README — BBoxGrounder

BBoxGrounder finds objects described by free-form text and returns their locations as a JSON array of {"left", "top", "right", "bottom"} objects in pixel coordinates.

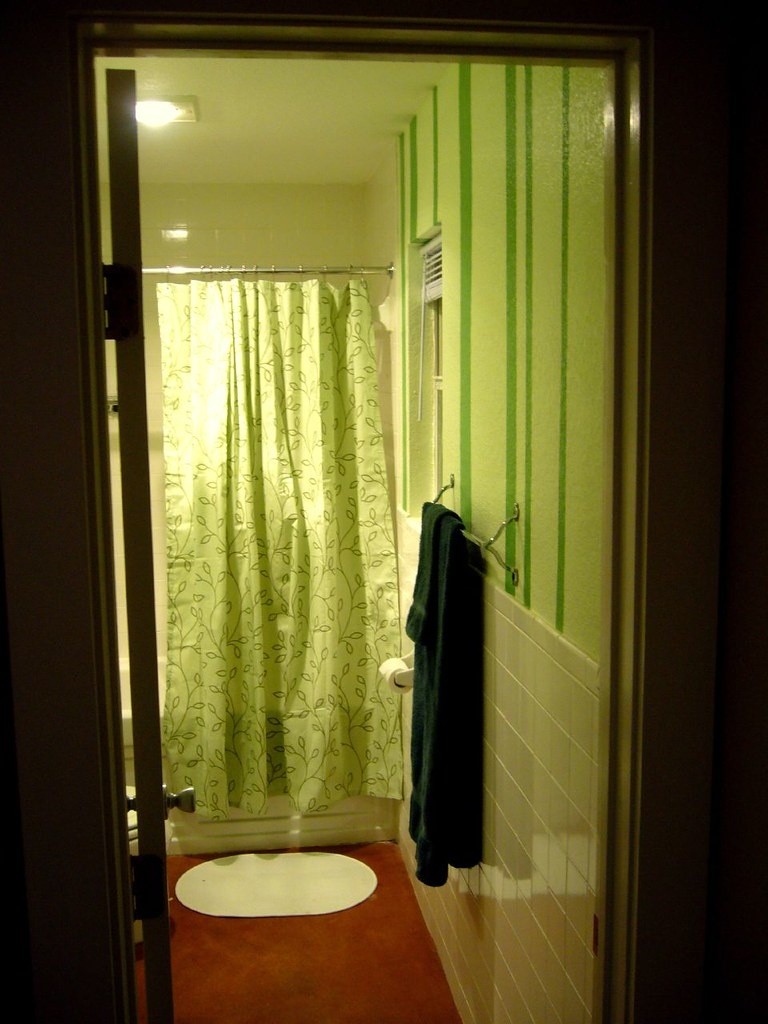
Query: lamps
[{"left": 135, "top": 96, "right": 200, "bottom": 132}]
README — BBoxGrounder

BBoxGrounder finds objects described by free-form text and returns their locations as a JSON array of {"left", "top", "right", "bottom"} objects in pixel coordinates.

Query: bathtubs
[{"left": 120, "top": 660, "right": 402, "bottom": 855}]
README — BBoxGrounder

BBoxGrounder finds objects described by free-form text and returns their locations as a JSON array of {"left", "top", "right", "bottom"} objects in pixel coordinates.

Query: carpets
[{"left": 174, "top": 852, "right": 378, "bottom": 917}]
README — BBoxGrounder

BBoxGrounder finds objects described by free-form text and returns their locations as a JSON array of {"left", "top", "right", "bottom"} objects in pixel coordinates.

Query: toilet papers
[{"left": 378, "top": 657, "right": 413, "bottom": 694}]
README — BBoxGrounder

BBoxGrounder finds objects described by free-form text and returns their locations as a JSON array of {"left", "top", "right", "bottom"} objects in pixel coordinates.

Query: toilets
[{"left": 127, "top": 782, "right": 173, "bottom": 946}]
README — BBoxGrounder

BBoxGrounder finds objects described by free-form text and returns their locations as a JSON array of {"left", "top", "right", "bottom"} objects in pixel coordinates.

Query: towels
[{"left": 404, "top": 502, "right": 487, "bottom": 888}]
